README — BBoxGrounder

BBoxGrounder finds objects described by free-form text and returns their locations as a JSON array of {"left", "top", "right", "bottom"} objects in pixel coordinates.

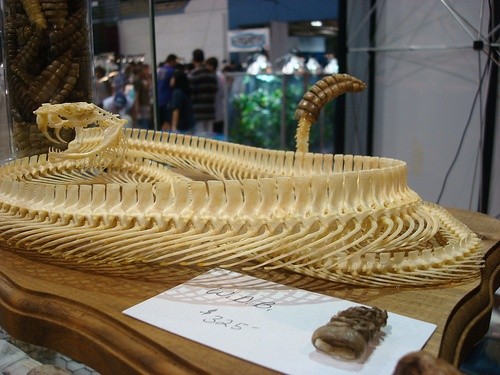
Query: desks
[{"left": 0, "top": 168, "right": 500, "bottom": 375}]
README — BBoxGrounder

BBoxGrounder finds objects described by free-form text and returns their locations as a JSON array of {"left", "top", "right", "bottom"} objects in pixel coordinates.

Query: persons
[
  {"left": 149, "top": 49, "right": 228, "bottom": 134},
  {"left": 94, "top": 64, "right": 154, "bottom": 130},
  {"left": 221, "top": 47, "right": 339, "bottom": 91}
]
